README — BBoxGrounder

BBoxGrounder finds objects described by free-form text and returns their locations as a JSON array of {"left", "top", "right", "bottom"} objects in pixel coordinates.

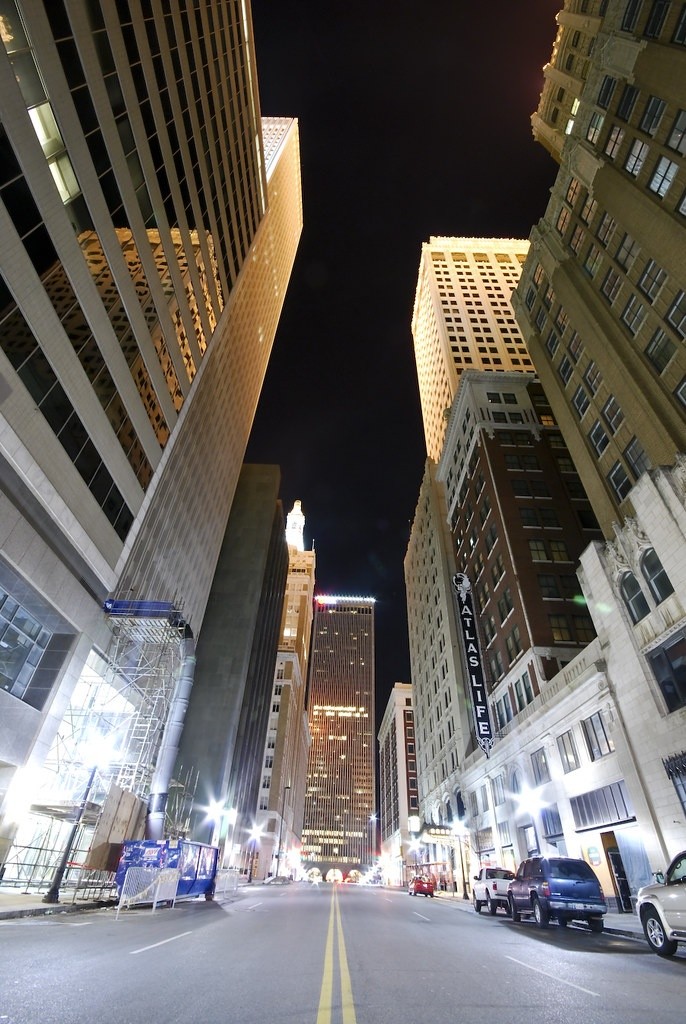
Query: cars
[
  {"left": 635, "top": 848, "right": 686, "bottom": 957},
  {"left": 407, "top": 876, "right": 434, "bottom": 897},
  {"left": 473, "top": 867, "right": 515, "bottom": 916},
  {"left": 505, "top": 856, "right": 608, "bottom": 933}
]
